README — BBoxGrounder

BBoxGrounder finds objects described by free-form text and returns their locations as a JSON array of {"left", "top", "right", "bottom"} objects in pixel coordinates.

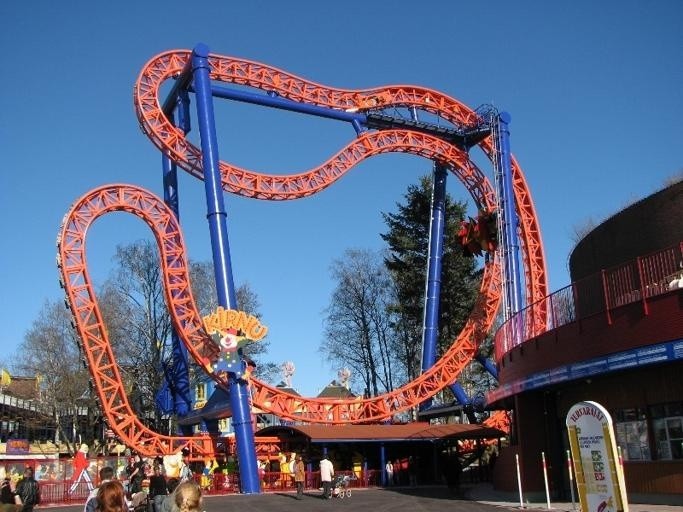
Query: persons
[
  {"left": 386, "top": 456, "right": 419, "bottom": 487},
  {"left": 440, "top": 452, "right": 464, "bottom": 500},
  {"left": 293, "top": 454, "right": 305, "bottom": 500},
  {"left": 0, "top": 464, "right": 41, "bottom": 510},
  {"left": 84, "top": 457, "right": 203, "bottom": 512},
  {"left": 319, "top": 454, "right": 334, "bottom": 500},
  {"left": 66, "top": 443, "right": 94, "bottom": 494}
]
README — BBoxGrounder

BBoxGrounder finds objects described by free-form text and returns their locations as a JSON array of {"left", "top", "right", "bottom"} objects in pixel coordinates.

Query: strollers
[{"left": 331, "top": 473, "right": 352, "bottom": 499}]
